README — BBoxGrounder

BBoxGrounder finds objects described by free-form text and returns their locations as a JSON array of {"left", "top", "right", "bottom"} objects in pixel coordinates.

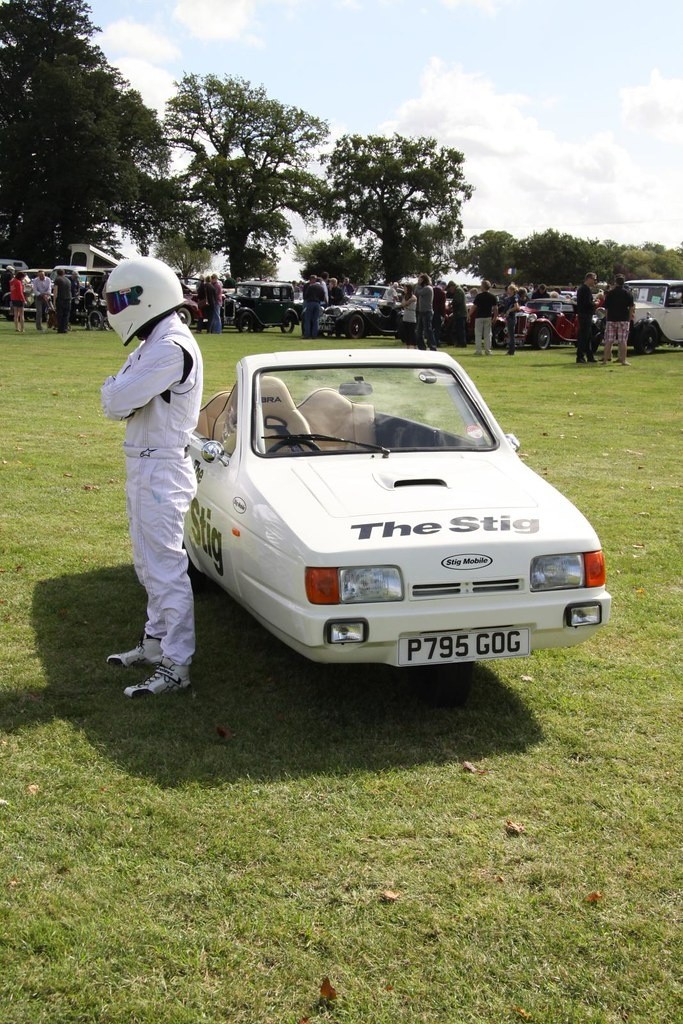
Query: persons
[
  {"left": 447, "top": 281, "right": 468, "bottom": 348},
  {"left": 386, "top": 281, "right": 399, "bottom": 301},
  {"left": 94, "top": 272, "right": 108, "bottom": 296},
  {"left": 401, "top": 282, "right": 417, "bottom": 349},
  {"left": 99, "top": 256, "right": 204, "bottom": 699},
  {"left": 84, "top": 280, "right": 101, "bottom": 330},
  {"left": 68, "top": 270, "right": 81, "bottom": 325},
  {"left": 291, "top": 272, "right": 355, "bottom": 338},
  {"left": 33, "top": 270, "right": 52, "bottom": 330},
  {"left": 600, "top": 274, "right": 634, "bottom": 366},
  {"left": 9, "top": 272, "right": 28, "bottom": 332},
  {"left": 1, "top": 266, "right": 15, "bottom": 293},
  {"left": 467, "top": 280, "right": 498, "bottom": 355},
  {"left": 415, "top": 274, "right": 438, "bottom": 351},
  {"left": 196, "top": 273, "right": 244, "bottom": 334},
  {"left": 53, "top": 269, "right": 70, "bottom": 333},
  {"left": 503, "top": 284, "right": 519, "bottom": 354},
  {"left": 433, "top": 281, "right": 449, "bottom": 347},
  {"left": 500, "top": 287, "right": 507, "bottom": 300},
  {"left": 523, "top": 282, "right": 612, "bottom": 298},
  {"left": 519, "top": 288, "right": 530, "bottom": 305},
  {"left": 576, "top": 272, "right": 600, "bottom": 364},
  {"left": 469, "top": 288, "right": 479, "bottom": 297}
]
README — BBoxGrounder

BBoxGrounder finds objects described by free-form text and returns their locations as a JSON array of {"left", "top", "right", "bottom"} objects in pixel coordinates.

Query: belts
[{"left": 122, "top": 444, "right": 190, "bottom": 460}]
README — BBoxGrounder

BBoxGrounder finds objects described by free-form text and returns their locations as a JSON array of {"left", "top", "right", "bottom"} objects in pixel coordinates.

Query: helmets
[{"left": 104, "top": 257, "right": 189, "bottom": 347}]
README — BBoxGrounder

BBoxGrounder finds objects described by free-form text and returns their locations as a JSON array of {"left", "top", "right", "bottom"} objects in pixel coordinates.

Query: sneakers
[
  {"left": 123, "top": 657, "right": 191, "bottom": 699},
  {"left": 106, "top": 632, "right": 163, "bottom": 667}
]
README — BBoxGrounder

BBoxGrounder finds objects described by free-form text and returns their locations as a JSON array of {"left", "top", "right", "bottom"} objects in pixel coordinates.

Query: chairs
[{"left": 193, "top": 375, "right": 377, "bottom": 455}]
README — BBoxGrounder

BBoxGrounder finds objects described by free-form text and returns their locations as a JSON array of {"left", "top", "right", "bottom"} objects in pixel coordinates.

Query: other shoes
[
  {"left": 406, "top": 342, "right": 466, "bottom": 351},
  {"left": 576, "top": 357, "right": 631, "bottom": 366},
  {"left": 506, "top": 351, "right": 514, "bottom": 355},
  {"left": 197, "top": 329, "right": 221, "bottom": 334},
  {"left": 15, "top": 326, "right": 68, "bottom": 334},
  {"left": 473, "top": 351, "right": 492, "bottom": 356}
]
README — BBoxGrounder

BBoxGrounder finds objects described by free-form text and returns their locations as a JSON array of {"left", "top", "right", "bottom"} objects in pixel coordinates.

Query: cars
[
  {"left": 589, "top": 279, "right": 683, "bottom": 354},
  {"left": 1, "top": 264, "right": 585, "bottom": 352},
  {"left": 180, "top": 348, "right": 611, "bottom": 708}
]
